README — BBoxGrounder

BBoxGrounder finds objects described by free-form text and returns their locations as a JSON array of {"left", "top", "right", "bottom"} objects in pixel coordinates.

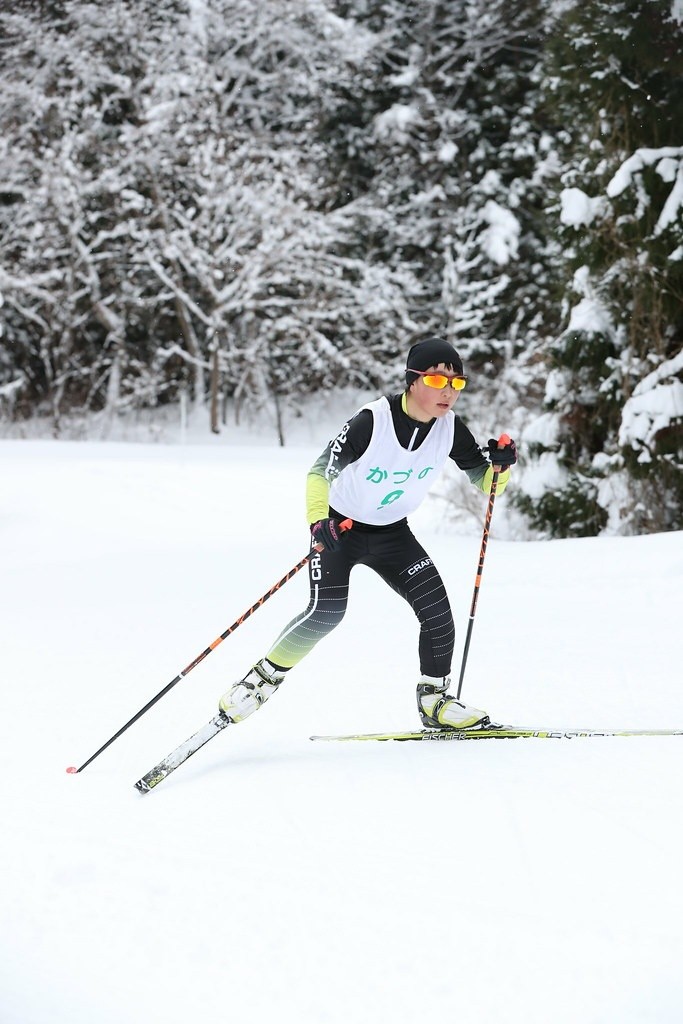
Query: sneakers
[
  {"left": 219, "top": 659, "right": 285, "bottom": 723},
  {"left": 416, "top": 675, "right": 491, "bottom": 731}
]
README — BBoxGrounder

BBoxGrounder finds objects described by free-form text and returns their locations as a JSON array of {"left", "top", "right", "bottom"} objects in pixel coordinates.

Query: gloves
[
  {"left": 481, "top": 439, "right": 518, "bottom": 472},
  {"left": 310, "top": 518, "right": 348, "bottom": 552}
]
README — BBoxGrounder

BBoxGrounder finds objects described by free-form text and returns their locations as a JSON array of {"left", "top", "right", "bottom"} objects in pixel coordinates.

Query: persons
[{"left": 220, "top": 335, "right": 524, "bottom": 733}]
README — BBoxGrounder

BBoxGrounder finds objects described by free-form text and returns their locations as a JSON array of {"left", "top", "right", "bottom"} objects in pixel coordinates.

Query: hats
[{"left": 405, "top": 338, "right": 462, "bottom": 387}]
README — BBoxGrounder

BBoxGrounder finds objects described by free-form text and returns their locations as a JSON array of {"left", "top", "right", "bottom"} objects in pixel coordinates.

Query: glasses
[{"left": 405, "top": 369, "right": 468, "bottom": 390}]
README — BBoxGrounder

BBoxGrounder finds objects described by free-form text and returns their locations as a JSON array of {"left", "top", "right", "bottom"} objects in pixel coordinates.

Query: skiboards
[{"left": 133, "top": 702, "right": 683, "bottom": 795}]
